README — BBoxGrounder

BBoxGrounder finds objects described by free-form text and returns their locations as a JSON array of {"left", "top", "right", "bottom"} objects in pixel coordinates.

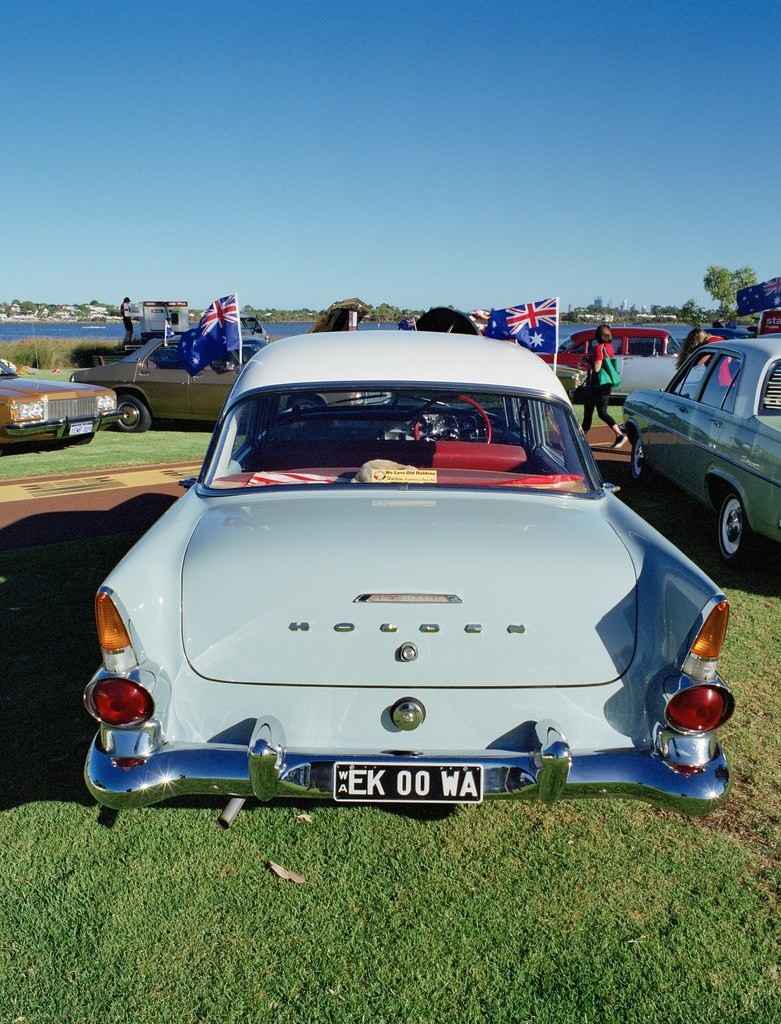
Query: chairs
[{"left": 425, "top": 441, "right": 526, "bottom": 471}]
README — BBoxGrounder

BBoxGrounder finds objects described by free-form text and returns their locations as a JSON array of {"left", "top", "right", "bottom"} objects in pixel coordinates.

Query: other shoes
[{"left": 611, "top": 435, "right": 628, "bottom": 449}]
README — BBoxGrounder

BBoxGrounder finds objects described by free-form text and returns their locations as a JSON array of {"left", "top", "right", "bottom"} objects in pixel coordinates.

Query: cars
[
  {"left": 70, "top": 334, "right": 393, "bottom": 434},
  {"left": 618, "top": 335, "right": 781, "bottom": 567},
  {"left": 0, "top": 362, "right": 123, "bottom": 449},
  {"left": 80, "top": 329, "right": 735, "bottom": 826},
  {"left": 536, "top": 322, "right": 709, "bottom": 402}
]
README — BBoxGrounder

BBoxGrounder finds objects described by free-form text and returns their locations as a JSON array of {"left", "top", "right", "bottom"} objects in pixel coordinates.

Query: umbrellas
[{"left": 416, "top": 306, "right": 481, "bottom": 335}]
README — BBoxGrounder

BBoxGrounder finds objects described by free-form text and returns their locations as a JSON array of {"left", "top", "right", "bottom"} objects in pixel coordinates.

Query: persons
[
  {"left": 121, "top": 297, "right": 133, "bottom": 346},
  {"left": 579, "top": 325, "right": 628, "bottom": 448},
  {"left": 677, "top": 327, "right": 735, "bottom": 389}
]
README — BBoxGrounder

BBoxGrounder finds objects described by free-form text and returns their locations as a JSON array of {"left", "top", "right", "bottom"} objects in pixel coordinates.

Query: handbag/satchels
[
  {"left": 573, "top": 384, "right": 588, "bottom": 405},
  {"left": 590, "top": 345, "right": 621, "bottom": 388}
]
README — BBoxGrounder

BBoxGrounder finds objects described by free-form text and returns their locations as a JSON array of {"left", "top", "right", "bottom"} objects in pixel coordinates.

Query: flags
[
  {"left": 397, "top": 317, "right": 415, "bottom": 330},
  {"left": 165, "top": 321, "right": 174, "bottom": 337},
  {"left": 484, "top": 298, "right": 557, "bottom": 354},
  {"left": 736, "top": 277, "right": 781, "bottom": 317},
  {"left": 177, "top": 295, "right": 240, "bottom": 378}
]
